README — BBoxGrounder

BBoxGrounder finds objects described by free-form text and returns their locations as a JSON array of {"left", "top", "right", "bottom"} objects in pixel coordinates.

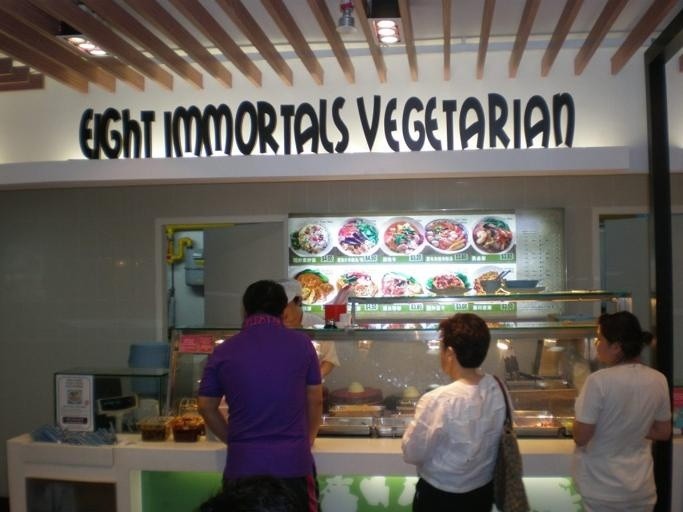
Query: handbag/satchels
[{"left": 491, "top": 421, "right": 530, "bottom": 511}]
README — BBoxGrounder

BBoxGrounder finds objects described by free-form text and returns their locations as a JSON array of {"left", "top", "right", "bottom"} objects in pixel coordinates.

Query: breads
[{"left": 295, "top": 271, "right": 333, "bottom": 305}]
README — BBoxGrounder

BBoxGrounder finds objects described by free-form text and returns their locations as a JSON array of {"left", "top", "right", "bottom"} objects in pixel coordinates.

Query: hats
[{"left": 277, "top": 278, "right": 303, "bottom": 304}]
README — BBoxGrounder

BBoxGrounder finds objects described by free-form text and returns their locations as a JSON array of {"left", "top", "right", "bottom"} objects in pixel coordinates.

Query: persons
[
  {"left": 570, "top": 310, "right": 672, "bottom": 512},
  {"left": 195, "top": 476, "right": 307, "bottom": 512},
  {"left": 311, "top": 340, "right": 341, "bottom": 378},
  {"left": 196, "top": 280, "right": 323, "bottom": 512},
  {"left": 401, "top": 313, "right": 515, "bottom": 512}
]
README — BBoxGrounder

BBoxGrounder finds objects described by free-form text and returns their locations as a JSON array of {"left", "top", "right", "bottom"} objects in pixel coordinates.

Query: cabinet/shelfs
[{"left": 7, "top": 291, "right": 636, "bottom": 512}]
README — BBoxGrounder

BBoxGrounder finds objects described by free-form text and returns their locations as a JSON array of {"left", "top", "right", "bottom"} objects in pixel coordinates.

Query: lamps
[
  {"left": 367, "top": 0, "right": 407, "bottom": 47},
  {"left": 56, "top": 22, "right": 107, "bottom": 59}
]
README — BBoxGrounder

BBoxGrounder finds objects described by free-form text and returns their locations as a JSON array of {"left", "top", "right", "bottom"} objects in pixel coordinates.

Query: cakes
[{"left": 299, "top": 224, "right": 329, "bottom": 253}]
